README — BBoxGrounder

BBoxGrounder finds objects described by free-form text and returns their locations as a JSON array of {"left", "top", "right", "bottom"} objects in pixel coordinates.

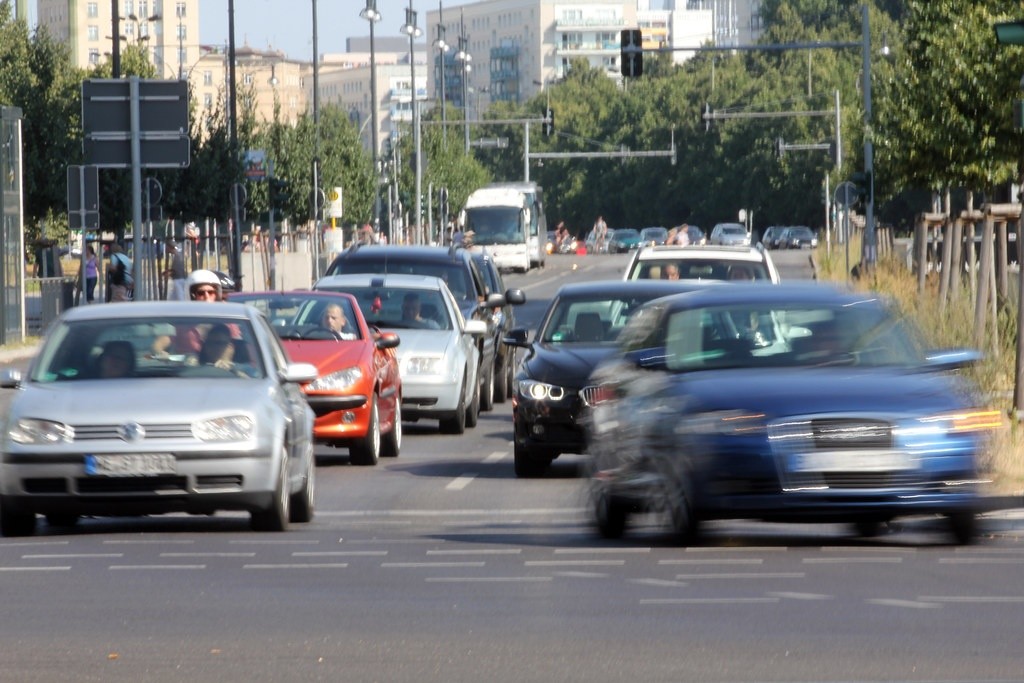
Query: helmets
[{"left": 186, "top": 270, "right": 222, "bottom": 302}]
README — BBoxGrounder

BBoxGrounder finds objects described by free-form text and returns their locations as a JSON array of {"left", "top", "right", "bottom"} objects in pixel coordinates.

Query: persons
[
  {"left": 594, "top": 216, "right": 607, "bottom": 243},
  {"left": 96, "top": 341, "right": 135, "bottom": 377},
  {"left": 660, "top": 263, "right": 680, "bottom": 280},
  {"left": 110, "top": 244, "right": 131, "bottom": 301},
  {"left": 161, "top": 241, "right": 186, "bottom": 300},
  {"left": 320, "top": 303, "right": 357, "bottom": 340},
  {"left": 86, "top": 245, "right": 99, "bottom": 304},
  {"left": 32, "top": 240, "right": 62, "bottom": 289},
  {"left": 152, "top": 269, "right": 242, "bottom": 355},
  {"left": 401, "top": 293, "right": 440, "bottom": 329},
  {"left": 199, "top": 324, "right": 248, "bottom": 378},
  {"left": 665, "top": 223, "right": 689, "bottom": 246}
]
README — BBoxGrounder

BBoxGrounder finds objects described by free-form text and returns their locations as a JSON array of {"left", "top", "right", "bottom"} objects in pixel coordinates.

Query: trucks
[{"left": 458, "top": 181, "right": 548, "bottom": 275}]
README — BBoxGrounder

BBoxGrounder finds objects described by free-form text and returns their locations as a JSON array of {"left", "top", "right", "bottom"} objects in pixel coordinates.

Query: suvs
[
  {"left": 325, "top": 239, "right": 525, "bottom": 415},
  {"left": 603, "top": 243, "right": 814, "bottom": 360}
]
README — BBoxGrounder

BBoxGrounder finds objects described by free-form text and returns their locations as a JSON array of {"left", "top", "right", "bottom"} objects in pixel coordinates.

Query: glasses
[{"left": 196, "top": 290, "right": 216, "bottom": 296}]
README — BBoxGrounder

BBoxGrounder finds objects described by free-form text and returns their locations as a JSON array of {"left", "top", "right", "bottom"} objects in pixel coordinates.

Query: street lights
[
  {"left": 128, "top": 13, "right": 161, "bottom": 48},
  {"left": 399, "top": 0, "right": 423, "bottom": 245},
  {"left": 359, "top": 0, "right": 382, "bottom": 244},
  {"left": 453, "top": 7, "right": 473, "bottom": 158},
  {"left": 432, "top": 1, "right": 450, "bottom": 246}
]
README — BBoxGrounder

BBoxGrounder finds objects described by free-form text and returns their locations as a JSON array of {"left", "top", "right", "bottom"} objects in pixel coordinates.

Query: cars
[
  {"left": 271, "top": 274, "right": 488, "bottom": 434},
  {"left": 546, "top": 222, "right": 819, "bottom": 257},
  {"left": 584, "top": 280, "right": 984, "bottom": 548},
  {"left": 499, "top": 277, "right": 740, "bottom": 479},
  {"left": 0, "top": 301, "right": 316, "bottom": 540},
  {"left": 227, "top": 288, "right": 404, "bottom": 467}
]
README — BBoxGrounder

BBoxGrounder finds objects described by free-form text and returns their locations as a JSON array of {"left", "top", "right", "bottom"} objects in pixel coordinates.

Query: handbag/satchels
[{"left": 110, "top": 253, "right": 126, "bottom": 286}]
